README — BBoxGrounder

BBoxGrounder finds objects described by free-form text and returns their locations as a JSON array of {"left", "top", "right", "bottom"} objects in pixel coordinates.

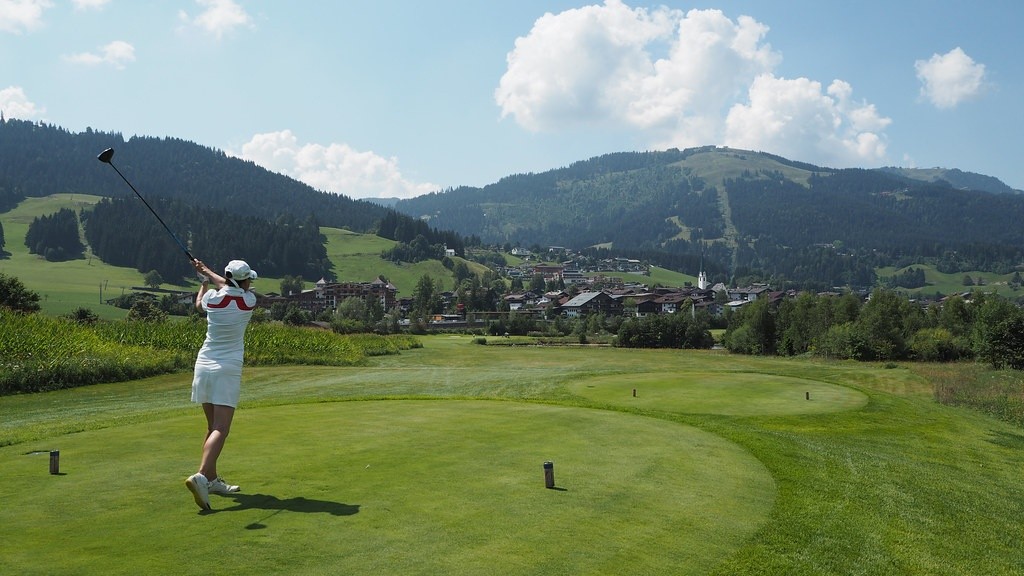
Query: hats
[{"left": 224, "top": 260, "right": 258, "bottom": 289}]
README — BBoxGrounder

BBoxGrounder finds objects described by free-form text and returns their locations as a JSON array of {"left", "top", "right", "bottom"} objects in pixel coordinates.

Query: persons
[{"left": 185, "top": 258, "right": 257, "bottom": 511}]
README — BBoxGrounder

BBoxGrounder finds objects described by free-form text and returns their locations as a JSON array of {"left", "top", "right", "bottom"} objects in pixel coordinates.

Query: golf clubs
[{"left": 96, "top": 147, "right": 196, "bottom": 262}]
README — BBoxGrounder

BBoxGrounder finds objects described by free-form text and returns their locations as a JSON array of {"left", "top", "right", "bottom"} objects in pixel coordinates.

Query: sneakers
[
  {"left": 185, "top": 473, "right": 211, "bottom": 510},
  {"left": 206, "top": 477, "right": 240, "bottom": 493}
]
